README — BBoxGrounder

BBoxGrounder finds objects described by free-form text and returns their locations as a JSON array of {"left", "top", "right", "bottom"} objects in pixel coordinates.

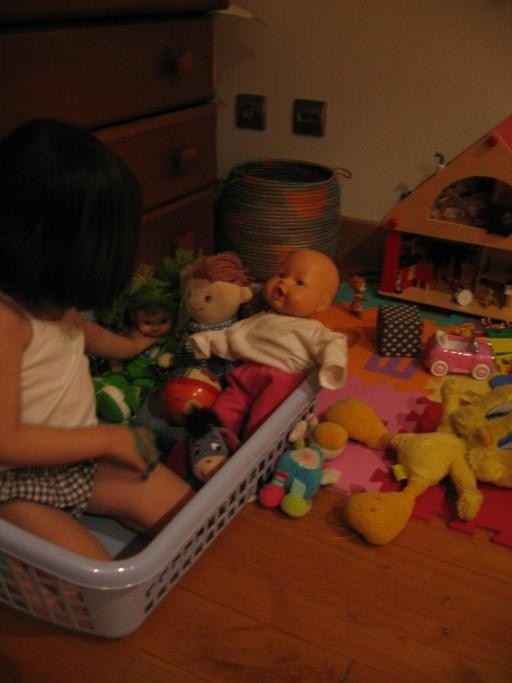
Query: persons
[
  {"left": 164, "top": 249, "right": 348, "bottom": 477},
  {"left": 90, "top": 246, "right": 254, "bottom": 485},
  {"left": 1, "top": 113, "right": 198, "bottom": 601}
]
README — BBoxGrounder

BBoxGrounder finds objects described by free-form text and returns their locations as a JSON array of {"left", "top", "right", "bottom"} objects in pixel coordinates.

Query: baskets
[{"left": 2, "top": 367, "right": 319, "bottom": 640}]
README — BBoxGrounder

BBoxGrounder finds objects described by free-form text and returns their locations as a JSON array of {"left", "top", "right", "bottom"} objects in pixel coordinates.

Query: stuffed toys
[
  {"left": 259, "top": 411, "right": 344, "bottom": 518},
  {"left": 349, "top": 174, "right": 512, "bottom": 381},
  {"left": 328, "top": 376, "right": 512, "bottom": 545}
]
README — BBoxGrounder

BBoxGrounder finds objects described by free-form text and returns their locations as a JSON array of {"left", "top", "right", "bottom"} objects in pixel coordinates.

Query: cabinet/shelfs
[{"left": 1, "top": 0, "right": 230, "bottom": 279}]
